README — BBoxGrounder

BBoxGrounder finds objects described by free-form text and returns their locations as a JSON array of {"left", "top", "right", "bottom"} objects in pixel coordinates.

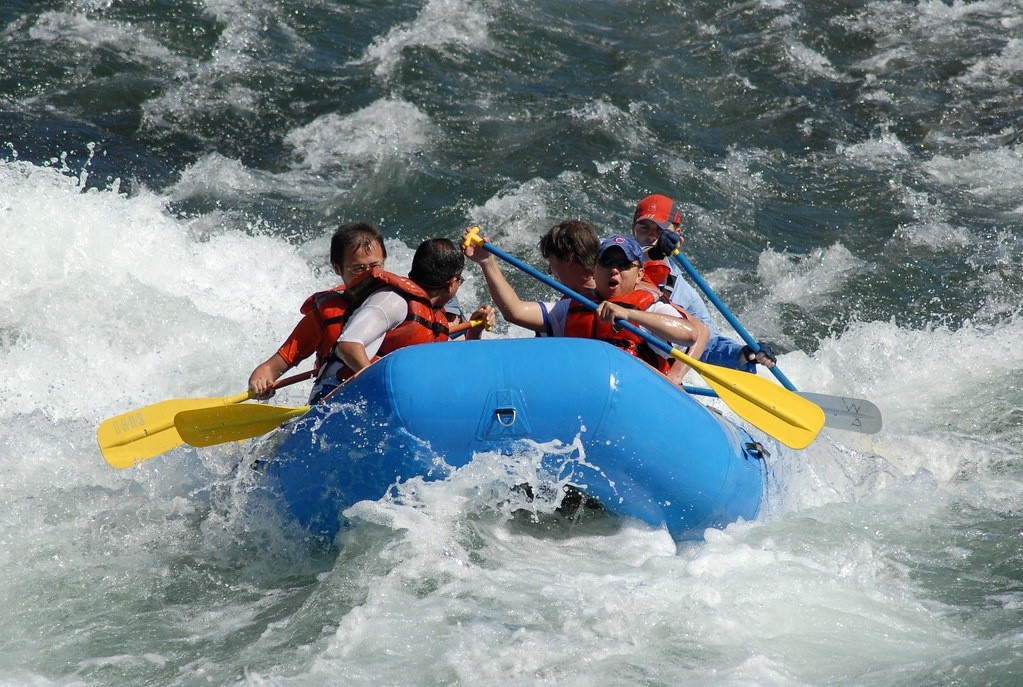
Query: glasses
[
  {"left": 455, "top": 275, "right": 465, "bottom": 286},
  {"left": 342, "top": 261, "right": 384, "bottom": 274},
  {"left": 598, "top": 255, "right": 642, "bottom": 270}
]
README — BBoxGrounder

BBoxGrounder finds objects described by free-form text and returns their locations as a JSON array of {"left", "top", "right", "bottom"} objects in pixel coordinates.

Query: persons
[
  {"left": 459, "top": 194, "right": 777, "bottom": 387},
  {"left": 247, "top": 221, "right": 496, "bottom": 407}
]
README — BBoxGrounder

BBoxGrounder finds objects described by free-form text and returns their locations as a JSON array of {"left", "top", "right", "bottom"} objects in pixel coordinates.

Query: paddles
[
  {"left": 681, "top": 385, "right": 882, "bottom": 434},
  {"left": 97, "top": 317, "right": 484, "bottom": 470},
  {"left": 463, "top": 226, "right": 825, "bottom": 450},
  {"left": 671, "top": 248, "right": 796, "bottom": 390},
  {"left": 174, "top": 403, "right": 314, "bottom": 448}
]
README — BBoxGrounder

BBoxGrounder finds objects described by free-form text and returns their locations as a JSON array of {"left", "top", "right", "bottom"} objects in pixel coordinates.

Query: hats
[
  {"left": 598, "top": 234, "right": 645, "bottom": 267},
  {"left": 442, "top": 295, "right": 461, "bottom": 315},
  {"left": 632, "top": 194, "right": 681, "bottom": 230}
]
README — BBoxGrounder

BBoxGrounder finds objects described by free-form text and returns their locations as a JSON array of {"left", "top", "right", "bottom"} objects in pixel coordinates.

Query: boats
[{"left": 251, "top": 339, "right": 775, "bottom": 560}]
